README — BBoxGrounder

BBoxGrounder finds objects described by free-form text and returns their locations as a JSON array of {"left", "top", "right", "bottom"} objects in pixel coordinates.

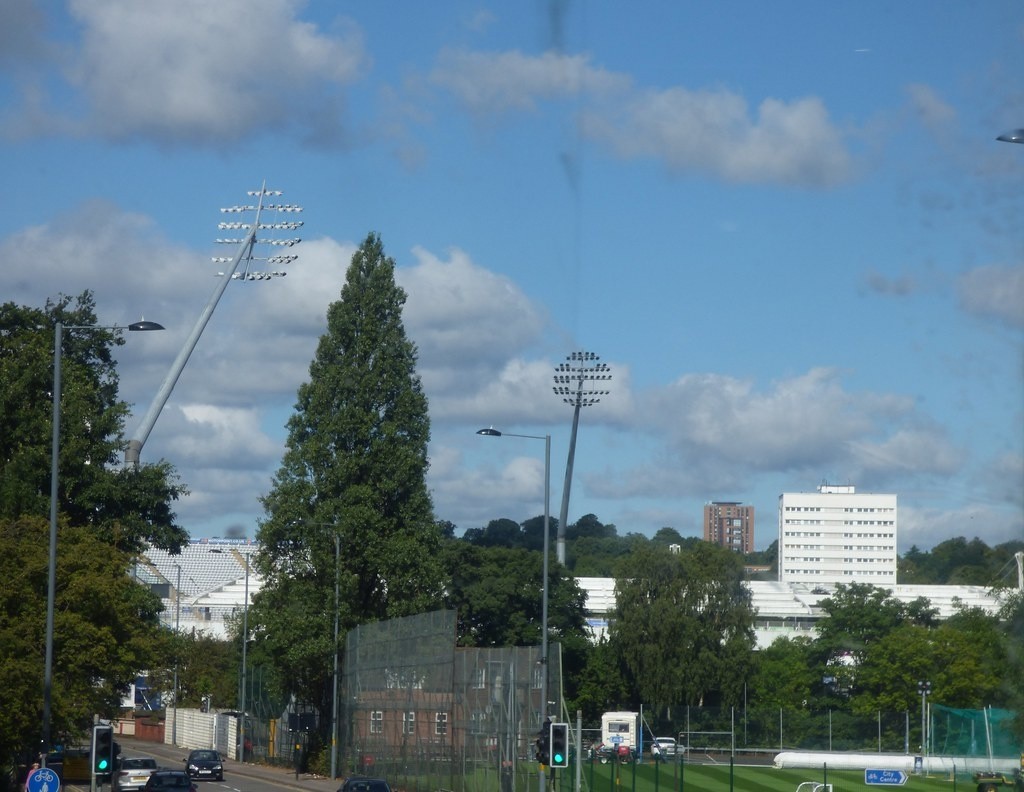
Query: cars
[
  {"left": 182, "top": 748, "right": 226, "bottom": 782},
  {"left": 111, "top": 757, "right": 160, "bottom": 792},
  {"left": 137, "top": 770, "right": 197, "bottom": 792},
  {"left": 337, "top": 777, "right": 393, "bottom": 792},
  {"left": 649, "top": 737, "right": 686, "bottom": 758}
]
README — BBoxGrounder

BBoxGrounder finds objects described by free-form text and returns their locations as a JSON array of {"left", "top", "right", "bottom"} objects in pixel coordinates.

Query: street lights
[
  {"left": 41, "top": 320, "right": 166, "bottom": 758},
  {"left": 206, "top": 548, "right": 251, "bottom": 767},
  {"left": 476, "top": 428, "right": 554, "bottom": 791}
]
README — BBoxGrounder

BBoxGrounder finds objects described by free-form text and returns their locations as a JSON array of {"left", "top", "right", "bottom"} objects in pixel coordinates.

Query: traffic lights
[
  {"left": 92, "top": 726, "right": 114, "bottom": 775},
  {"left": 548, "top": 722, "right": 569, "bottom": 770},
  {"left": 535, "top": 719, "right": 552, "bottom": 765}
]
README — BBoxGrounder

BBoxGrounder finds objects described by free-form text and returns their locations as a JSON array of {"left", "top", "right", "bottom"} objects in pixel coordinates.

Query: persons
[{"left": 25, "top": 763, "right": 40, "bottom": 792}]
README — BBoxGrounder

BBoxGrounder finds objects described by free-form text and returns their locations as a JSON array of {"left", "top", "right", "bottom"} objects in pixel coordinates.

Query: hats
[{"left": 32, "top": 763, "right": 40, "bottom": 767}]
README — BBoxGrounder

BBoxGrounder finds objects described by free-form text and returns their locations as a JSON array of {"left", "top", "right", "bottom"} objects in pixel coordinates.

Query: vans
[{"left": 601, "top": 710, "right": 643, "bottom": 759}]
